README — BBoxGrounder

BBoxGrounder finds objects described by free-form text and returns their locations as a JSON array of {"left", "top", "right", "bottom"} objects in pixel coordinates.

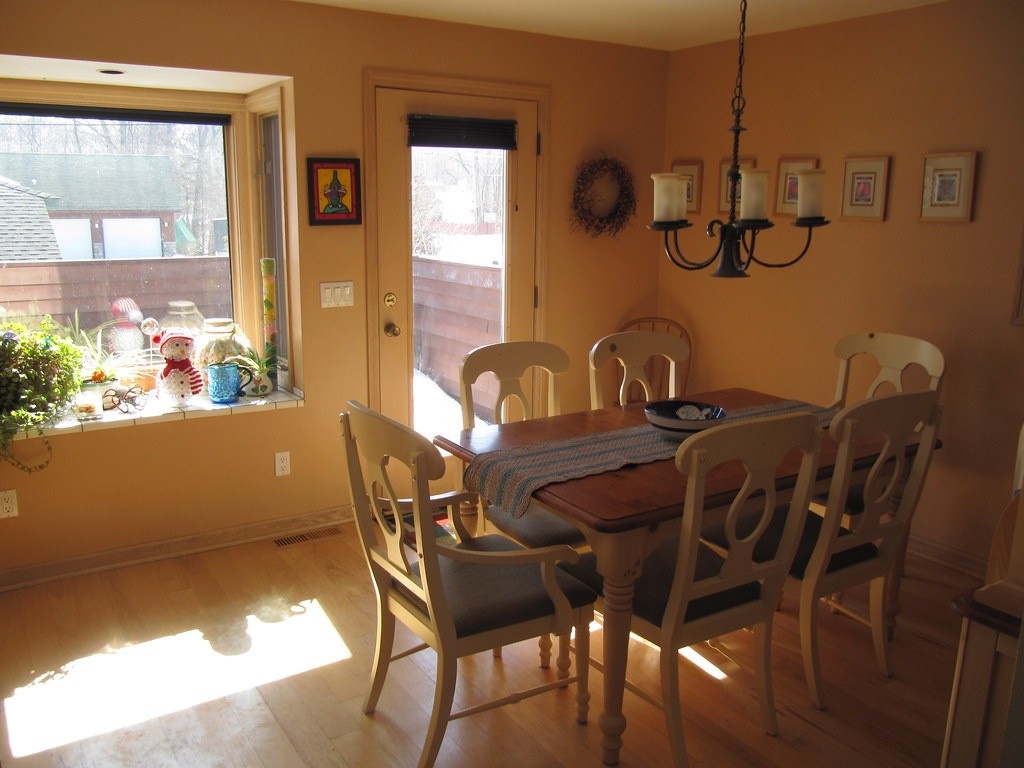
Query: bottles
[
  {"left": 152, "top": 301, "right": 206, "bottom": 356},
  {"left": 194, "top": 318, "right": 251, "bottom": 392}
]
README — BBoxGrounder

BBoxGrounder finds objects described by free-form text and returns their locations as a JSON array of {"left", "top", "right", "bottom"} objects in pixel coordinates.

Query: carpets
[{"left": 372, "top": 506, "right": 459, "bottom": 553}]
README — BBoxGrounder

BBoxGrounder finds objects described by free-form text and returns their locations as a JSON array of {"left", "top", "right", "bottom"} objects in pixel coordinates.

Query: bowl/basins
[{"left": 644, "top": 401, "right": 726, "bottom": 441}]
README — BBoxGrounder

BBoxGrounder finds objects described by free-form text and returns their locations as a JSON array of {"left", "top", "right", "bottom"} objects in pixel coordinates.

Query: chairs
[
  {"left": 697, "top": 390, "right": 944, "bottom": 709},
  {"left": 588, "top": 331, "right": 690, "bottom": 409},
  {"left": 613, "top": 317, "right": 691, "bottom": 406},
  {"left": 774, "top": 332, "right": 947, "bottom": 640},
  {"left": 459, "top": 341, "right": 589, "bottom": 549},
  {"left": 556, "top": 411, "right": 824, "bottom": 768}
]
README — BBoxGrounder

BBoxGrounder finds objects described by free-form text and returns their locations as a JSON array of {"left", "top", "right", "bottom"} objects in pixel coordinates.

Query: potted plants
[
  {"left": 223, "top": 344, "right": 282, "bottom": 396},
  {"left": 0, "top": 313, "right": 83, "bottom": 472},
  {"left": 80, "top": 373, "right": 119, "bottom": 409},
  {"left": 338, "top": 400, "right": 598, "bottom": 768}
]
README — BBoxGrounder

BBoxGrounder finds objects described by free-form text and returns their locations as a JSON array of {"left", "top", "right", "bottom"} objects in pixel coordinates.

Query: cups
[
  {"left": 75, "top": 391, "right": 103, "bottom": 421},
  {"left": 205, "top": 363, "right": 252, "bottom": 403}
]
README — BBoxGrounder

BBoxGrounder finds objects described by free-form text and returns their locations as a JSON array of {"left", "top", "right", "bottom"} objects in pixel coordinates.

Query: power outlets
[
  {"left": 275, "top": 451, "right": 291, "bottom": 477},
  {"left": 0, "top": 489, "right": 18, "bottom": 519}
]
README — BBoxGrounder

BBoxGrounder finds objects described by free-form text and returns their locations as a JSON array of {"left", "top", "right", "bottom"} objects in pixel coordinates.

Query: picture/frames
[
  {"left": 838, "top": 157, "right": 891, "bottom": 222},
  {"left": 646, "top": 0, "right": 831, "bottom": 277},
  {"left": 917, "top": 152, "right": 977, "bottom": 221},
  {"left": 671, "top": 159, "right": 704, "bottom": 215},
  {"left": 773, "top": 157, "right": 819, "bottom": 218},
  {"left": 717, "top": 159, "right": 756, "bottom": 215},
  {"left": 307, "top": 157, "right": 362, "bottom": 226}
]
